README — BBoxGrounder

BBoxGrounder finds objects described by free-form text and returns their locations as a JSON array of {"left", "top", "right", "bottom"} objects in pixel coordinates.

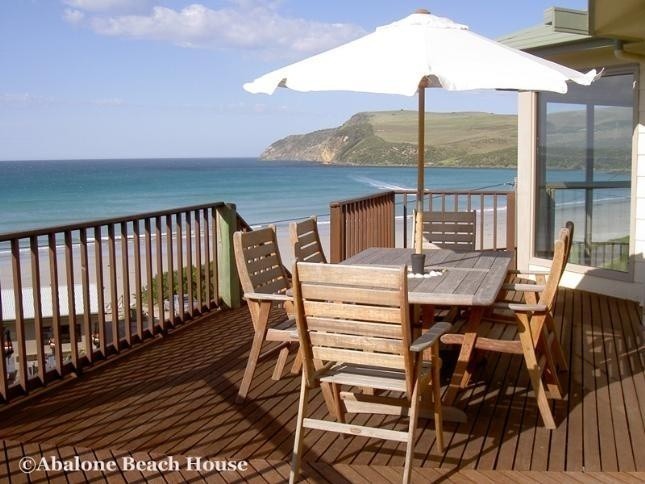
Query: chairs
[{"left": 233, "top": 207, "right": 578, "bottom": 484}]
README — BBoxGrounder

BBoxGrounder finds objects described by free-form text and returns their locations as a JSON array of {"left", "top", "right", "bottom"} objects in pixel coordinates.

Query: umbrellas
[{"left": 242, "top": 7, "right": 605, "bottom": 273}]
